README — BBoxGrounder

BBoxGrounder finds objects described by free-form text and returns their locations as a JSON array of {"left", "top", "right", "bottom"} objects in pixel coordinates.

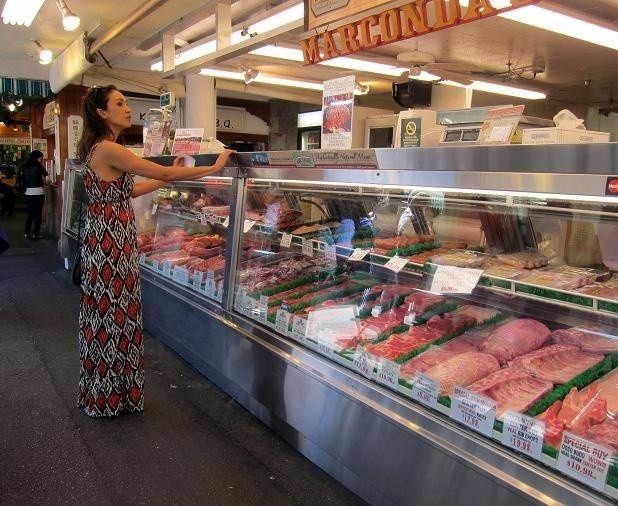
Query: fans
[{"left": 368, "top": 39, "right": 480, "bottom": 87}]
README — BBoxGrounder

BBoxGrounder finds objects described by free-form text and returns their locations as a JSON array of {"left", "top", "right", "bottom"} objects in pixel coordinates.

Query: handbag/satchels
[
  {"left": 71, "top": 247, "right": 83, "bottom": 286},
  {"left": 15, "top": 176, "right": 26, "bottom": 192}
]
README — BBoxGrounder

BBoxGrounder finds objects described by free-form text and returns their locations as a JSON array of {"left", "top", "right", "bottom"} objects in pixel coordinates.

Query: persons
[
  {"left": 23, "top": 150, "right": 48, "bottom": 242},
  {"left": 73, "top": 85, "right": 239, "bottom": 417}
]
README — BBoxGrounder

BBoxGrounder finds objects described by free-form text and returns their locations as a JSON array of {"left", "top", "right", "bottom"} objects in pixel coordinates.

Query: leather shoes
[{"left": 24, "top": 232, "right": 39, "bottom": 239}]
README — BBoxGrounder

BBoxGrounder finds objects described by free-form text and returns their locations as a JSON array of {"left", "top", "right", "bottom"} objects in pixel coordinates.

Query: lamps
[{"left": 54, "top": 0, "right": 81, "bottom": 32}]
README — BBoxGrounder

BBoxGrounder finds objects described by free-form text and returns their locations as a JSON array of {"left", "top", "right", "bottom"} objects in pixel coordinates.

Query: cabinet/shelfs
[{"left": 58, "top": 143, "right": 618, "bottom": 506}]
